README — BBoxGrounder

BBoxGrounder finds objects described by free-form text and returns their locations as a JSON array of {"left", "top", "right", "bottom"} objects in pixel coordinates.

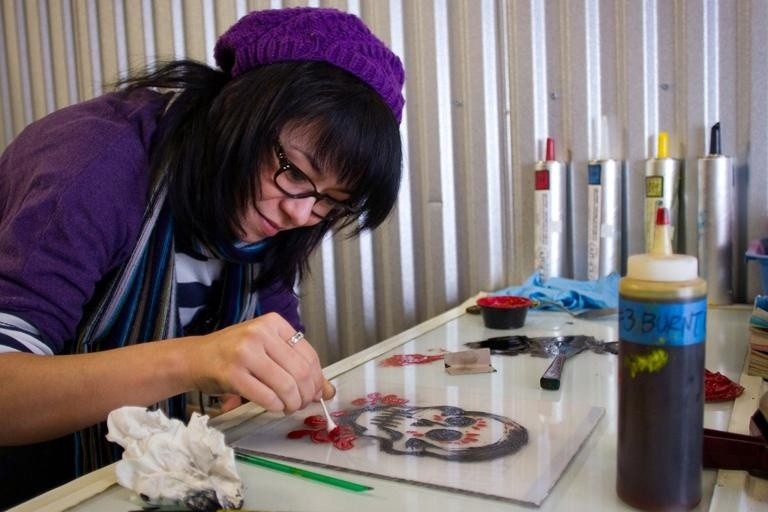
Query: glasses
[{"left": 274, "top": 139, "right": 361, "bottom": 222}]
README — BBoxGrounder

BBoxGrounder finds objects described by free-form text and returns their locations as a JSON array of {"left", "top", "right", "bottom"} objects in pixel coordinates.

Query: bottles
[
  {"left": 613, "top": 210, "right": 707, "bottom": 510},
  {"left": 536, "top": 116, "right": 734, "bottom": 307}
]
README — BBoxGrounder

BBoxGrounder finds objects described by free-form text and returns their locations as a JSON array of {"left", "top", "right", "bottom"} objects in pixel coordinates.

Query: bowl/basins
[{"left": 477, "top": 295, "right": 531, "bottom": 328}]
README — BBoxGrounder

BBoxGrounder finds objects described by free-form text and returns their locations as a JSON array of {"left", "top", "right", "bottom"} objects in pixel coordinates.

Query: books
[{"left": 747, "top": 294, "right": 768, "bottom": 380}]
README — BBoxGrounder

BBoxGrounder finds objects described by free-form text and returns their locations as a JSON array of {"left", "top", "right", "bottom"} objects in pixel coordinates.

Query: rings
[{"left": 288, "top": 330, "right": 306, "bottom": 347}]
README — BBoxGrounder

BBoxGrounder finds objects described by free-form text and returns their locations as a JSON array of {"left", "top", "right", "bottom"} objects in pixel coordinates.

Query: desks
[{"left": 1, "top": 290, "right": 768, "bottom": 511}]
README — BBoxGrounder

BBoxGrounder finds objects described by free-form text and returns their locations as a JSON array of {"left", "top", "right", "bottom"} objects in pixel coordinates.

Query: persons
[{"left": 1, "top": 7, "right": 406, "bottom": 512}]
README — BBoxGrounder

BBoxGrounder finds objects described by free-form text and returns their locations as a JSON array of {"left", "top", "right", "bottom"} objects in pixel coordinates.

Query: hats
[{"left": 215, "top": 9, "right": 405, "bottom": 126}]
